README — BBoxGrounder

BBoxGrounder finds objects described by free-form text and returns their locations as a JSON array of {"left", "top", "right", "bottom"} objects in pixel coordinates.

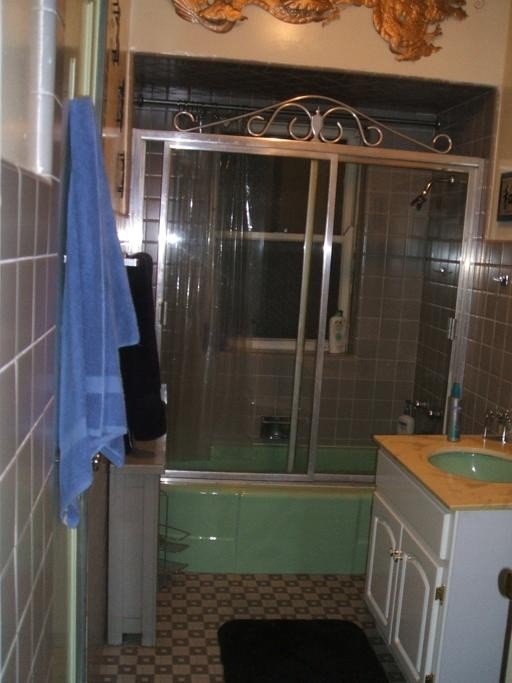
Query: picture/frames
[{"left": 496, "top": 172, "right": 512, "bottom": 221}]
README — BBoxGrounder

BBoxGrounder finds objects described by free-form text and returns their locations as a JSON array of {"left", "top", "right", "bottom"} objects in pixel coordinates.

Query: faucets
[
  {"left": 480, "top": 404, "right": 512, "bottom": 445},
  {"left": 411, "top": 399, "right": 433, "bottom": 419}
]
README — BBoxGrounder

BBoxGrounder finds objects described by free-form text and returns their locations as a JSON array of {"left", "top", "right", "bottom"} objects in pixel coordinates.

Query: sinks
[{"left": 427, "top": 446, "right": 512, "bottom": 486}]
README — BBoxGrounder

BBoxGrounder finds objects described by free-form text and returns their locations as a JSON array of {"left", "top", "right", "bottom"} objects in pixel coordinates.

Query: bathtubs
[{"left": 158, "top": 438, "right": 377, "bottom": 579}]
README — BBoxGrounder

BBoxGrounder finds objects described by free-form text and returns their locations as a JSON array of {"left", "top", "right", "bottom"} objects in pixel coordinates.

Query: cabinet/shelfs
[
  {"left": 217, "top": 619, "right": 390, "bottom": 683},
  {"left": 361, "top": 450, "right": 512, "bottom": 683}
]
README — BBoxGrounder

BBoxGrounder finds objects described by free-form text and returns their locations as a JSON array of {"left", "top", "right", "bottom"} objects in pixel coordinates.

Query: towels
[{"left": 57, "top": 97, "right": 142, "bottom": 526}]
